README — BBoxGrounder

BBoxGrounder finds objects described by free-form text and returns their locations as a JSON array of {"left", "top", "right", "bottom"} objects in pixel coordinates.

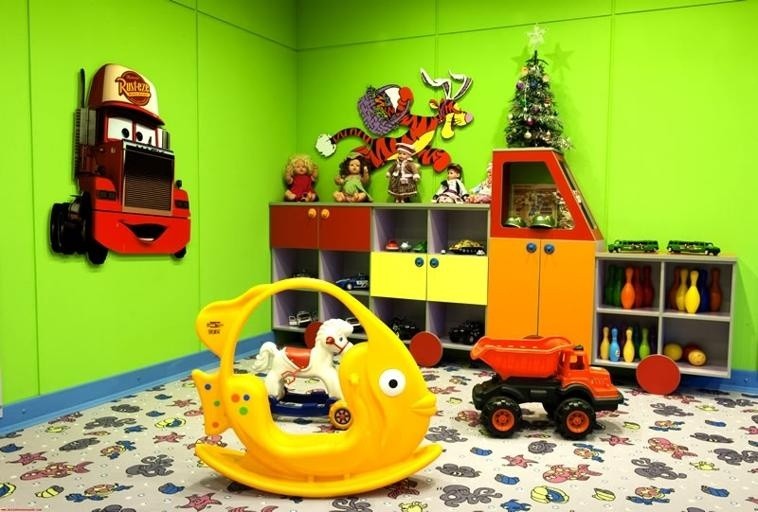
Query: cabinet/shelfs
[
  {"left": 487, "top": 145, "right": 604, "bottom": 366},
  {"left": 269, "top": 200, "right": 489, "bottom": 360},
  {"left": 594, "top": 258, "right": 736, "bottom": 381}
]
